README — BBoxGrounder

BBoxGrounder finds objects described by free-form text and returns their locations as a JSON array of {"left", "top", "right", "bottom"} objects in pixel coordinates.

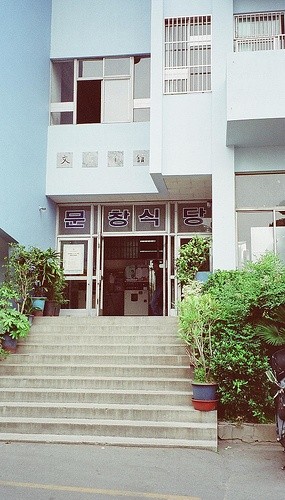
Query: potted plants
[
  {"left": 8, "top": 242, "right": 57, "bottom": 316},
  {"left": 172, "top": 294, "right": 220, "bottom": 412},
  {"left": 44, "top": 248, "right": 64, "bottom": 316},
  {"left": 0, "top": 281, "right": 31, "bottom": 354},
  {"left": 54, "top": 268, "right": 70, "bottom": 316}
]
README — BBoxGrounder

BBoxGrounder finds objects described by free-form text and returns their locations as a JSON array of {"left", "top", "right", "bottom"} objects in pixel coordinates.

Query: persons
[{"left": 149, "top": 259, "right": 163, "bottom": 315}]
